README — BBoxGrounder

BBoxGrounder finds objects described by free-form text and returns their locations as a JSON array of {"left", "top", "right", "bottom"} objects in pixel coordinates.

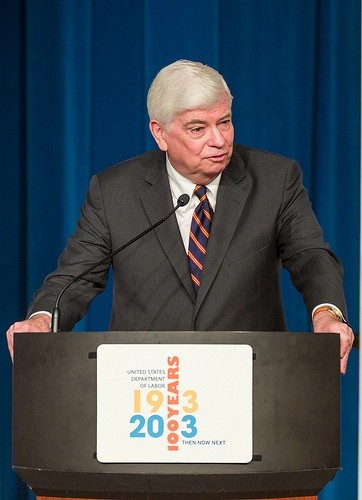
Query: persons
[{"left": 6, "top": 58, "right": 355, "bottom": 372}]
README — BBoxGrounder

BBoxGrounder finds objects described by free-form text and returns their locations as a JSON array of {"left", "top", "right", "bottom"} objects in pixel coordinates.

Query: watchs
[{"left": 313, "top": 306, "right": 343, "bottom": 322}]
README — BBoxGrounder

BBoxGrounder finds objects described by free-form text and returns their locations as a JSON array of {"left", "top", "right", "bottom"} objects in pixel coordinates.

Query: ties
[{"left": 187, "top": 184, "right": 215, "bottom": 300}]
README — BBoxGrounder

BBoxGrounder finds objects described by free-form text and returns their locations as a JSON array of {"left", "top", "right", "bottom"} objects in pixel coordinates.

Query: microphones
[{"left": 51, "top": 194, "right": 190, "bottom": 333}]
